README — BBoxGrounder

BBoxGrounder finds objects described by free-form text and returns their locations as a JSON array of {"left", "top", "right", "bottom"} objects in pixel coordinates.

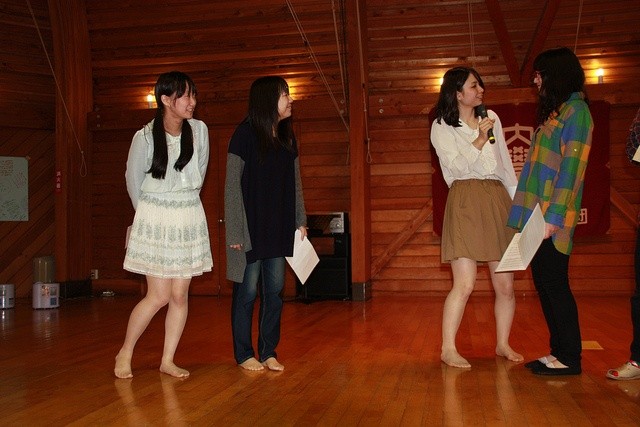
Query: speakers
[{"left": 295, "top": 233, "right": 351, "bottom": 303}]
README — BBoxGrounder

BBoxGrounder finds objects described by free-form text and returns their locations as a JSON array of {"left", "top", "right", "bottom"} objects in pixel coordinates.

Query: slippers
[{"left": 606, "top": 361, "right": 640, "bottom": 380}]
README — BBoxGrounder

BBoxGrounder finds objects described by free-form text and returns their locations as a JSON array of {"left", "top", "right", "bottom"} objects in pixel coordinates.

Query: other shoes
[{"left": 524, "top": 359, "right": 582, "bottom": 375}]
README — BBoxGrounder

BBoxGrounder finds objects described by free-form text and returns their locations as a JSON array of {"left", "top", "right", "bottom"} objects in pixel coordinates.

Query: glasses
[{"left": 532, "top": 71, "right": 540, "bottom": 78}]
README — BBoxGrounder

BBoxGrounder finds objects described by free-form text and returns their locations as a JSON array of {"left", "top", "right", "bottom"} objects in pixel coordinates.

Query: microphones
[{"left": 477, "top": 105, "right": 495, "bottom": 144}]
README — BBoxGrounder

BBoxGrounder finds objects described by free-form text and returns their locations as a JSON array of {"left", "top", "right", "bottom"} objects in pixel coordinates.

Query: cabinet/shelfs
[{"left": 295, "top": 232, "right": 349, "bottom": 302}]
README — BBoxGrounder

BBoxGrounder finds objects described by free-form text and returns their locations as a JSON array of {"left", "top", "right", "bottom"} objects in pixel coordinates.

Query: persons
[
  {"left": 224, "top": 76, "right": 307, "bottom": 371},
  {"left": 604, "top": 108, "right": 640, "bottom": 380},
  {"left": 114, "top": 70, "right": 214, "bottom": 379},
  {"left": 430, "top": 67, "right": 523, "bottom": 370},
  {"left": 507, "top": 46, "right": 594, "bottom": 374}
]
